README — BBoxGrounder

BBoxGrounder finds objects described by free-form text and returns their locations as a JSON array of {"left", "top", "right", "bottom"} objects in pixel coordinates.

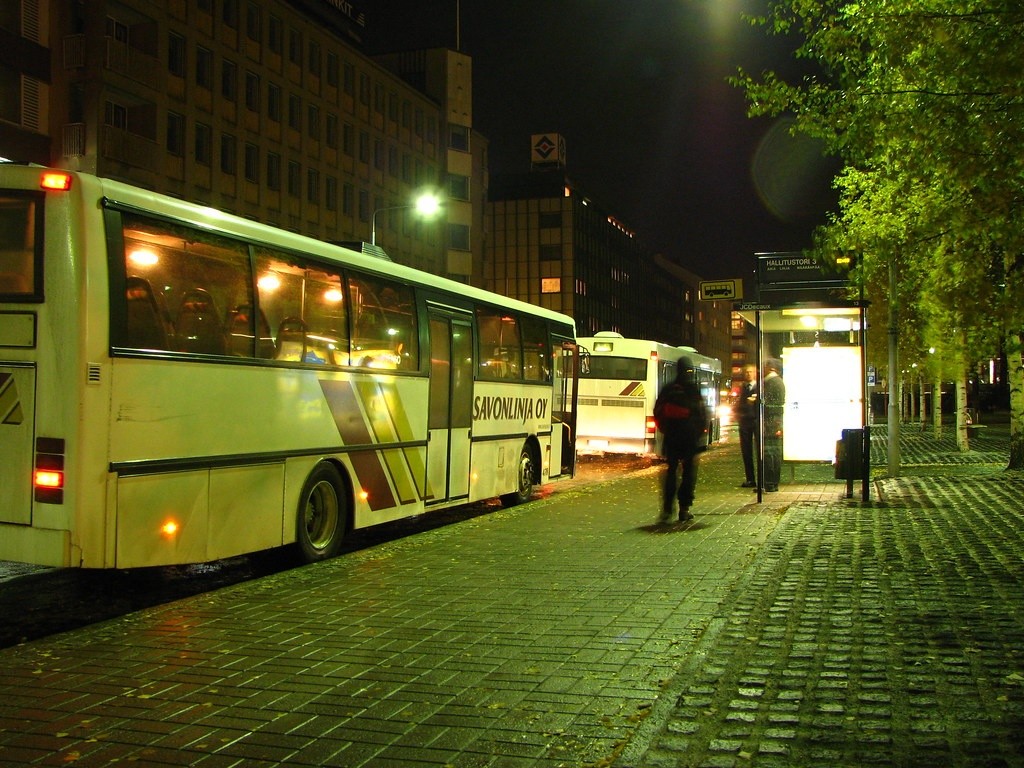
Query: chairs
[
  {"left": 126, "top": 275, "right": 175, "bottom": 352},
  {"left": 277, "top": 317, "right": 306, "bottom": 333},
  {"left": 222, "top": 304, "right": 279, "bottom": 360},
  {"left": 170, "top": 288, "right": 233, "bottom": 356}
]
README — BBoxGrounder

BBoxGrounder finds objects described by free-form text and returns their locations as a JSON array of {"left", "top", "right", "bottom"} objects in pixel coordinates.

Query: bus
[
  {"left": 554, "top": 331, "right": 722, "bottom": 463},
  {"left": 0, "top": 158, "right": 593, "bottom": 571}
]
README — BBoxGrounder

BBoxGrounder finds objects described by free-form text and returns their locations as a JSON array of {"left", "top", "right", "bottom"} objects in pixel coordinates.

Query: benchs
[
  {"left": 275, "top": 329, "right": 415, "bottom": 370},
  {"left": 834, "top": 459, "right": 863, "bottom": 497}
]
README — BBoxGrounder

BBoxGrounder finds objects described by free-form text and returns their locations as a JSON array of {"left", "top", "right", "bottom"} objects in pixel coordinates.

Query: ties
[{"left": 747, "top": 383, "right": 752, "bottom": 392}]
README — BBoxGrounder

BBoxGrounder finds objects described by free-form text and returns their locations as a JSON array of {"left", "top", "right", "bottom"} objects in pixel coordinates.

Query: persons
[
  {"left": 653, "top": 357, "right": 708, "bottom": 526},
  {"left": 735, "top": 359, "right": 785, "bottom": 492}
]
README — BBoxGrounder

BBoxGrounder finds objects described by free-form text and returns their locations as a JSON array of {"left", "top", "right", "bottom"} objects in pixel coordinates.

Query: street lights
[{"left": 368, "top": 180, "right": 442, "bottom": 245}]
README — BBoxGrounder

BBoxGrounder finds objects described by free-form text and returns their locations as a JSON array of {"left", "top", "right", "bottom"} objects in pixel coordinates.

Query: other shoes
[
  {"left": 677, "top": 510, "right": 693, "bottom": 521},
  {"left": 741, "top": 481, "right": 756, "bottom": 487},
  {"left": 660, "top": 513, "right": 674, "bottom": 524}
]
